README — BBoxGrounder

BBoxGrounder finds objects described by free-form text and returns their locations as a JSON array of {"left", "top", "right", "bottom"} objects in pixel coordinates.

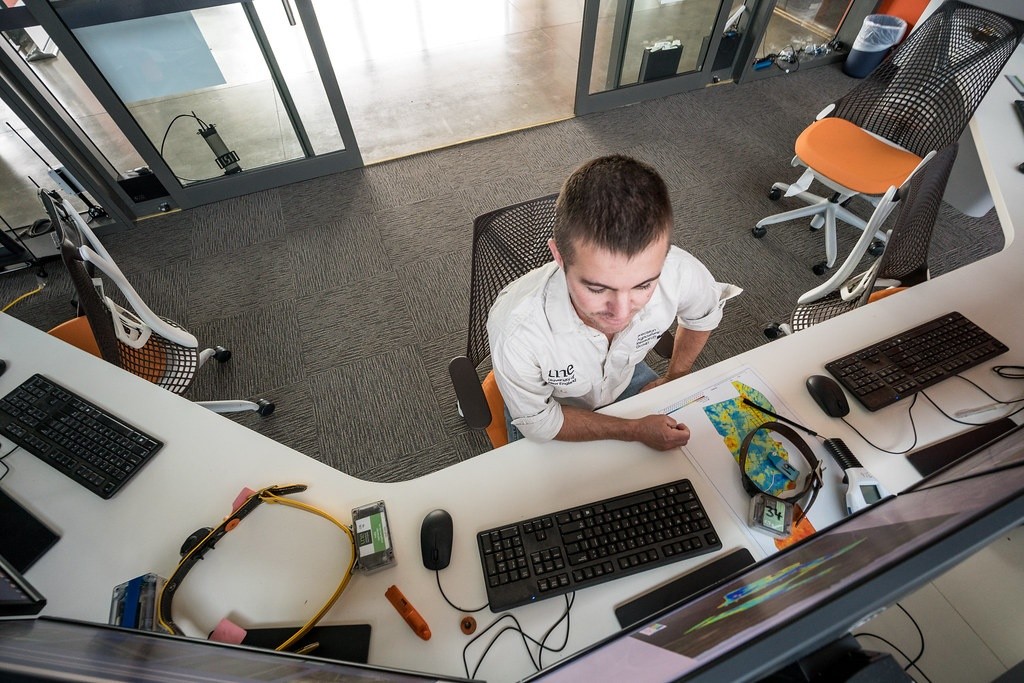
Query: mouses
[
  {"left": 0, "top": 358, "right": 6, "bottom": 375},
  {"left": 420, "top": 509, "right": 454, "bottom": 571},
  {"left": 805, "top": 374, "right": 850, "bottom": 418}
]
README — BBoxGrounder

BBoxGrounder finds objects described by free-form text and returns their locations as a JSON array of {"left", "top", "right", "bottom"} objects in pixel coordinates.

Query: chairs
[
  {"left": 764, "top": 140, "right": 959, "bottom": 340},
  {"left": 449, "top": 192, "right": 674, "bottom": 447},
  {"left": 752, "top": 0, "right": 1024, "bottom": 278},
  {"left": 39, "top": 187, "right": 276, "bottom": 419}
]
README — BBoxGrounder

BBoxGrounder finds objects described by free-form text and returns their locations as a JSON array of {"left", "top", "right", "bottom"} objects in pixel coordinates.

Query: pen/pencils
[{"left": 295, "top": 641, "right": 320, "bottom": 655}]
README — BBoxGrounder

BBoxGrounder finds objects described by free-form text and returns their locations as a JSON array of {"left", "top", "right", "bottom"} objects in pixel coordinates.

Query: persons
[{"left": 485, "top": 153, "right": 745, "bottom": 452}]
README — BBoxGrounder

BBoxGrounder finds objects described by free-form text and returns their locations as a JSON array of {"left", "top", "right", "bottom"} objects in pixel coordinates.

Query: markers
[{"left": 383, "top": 584, "right": 433, "bottom": 643}]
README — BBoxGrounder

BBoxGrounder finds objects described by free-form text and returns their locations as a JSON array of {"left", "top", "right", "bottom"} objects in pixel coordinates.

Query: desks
[
  {"left": 736, "top": 19, "right": 1024, "bottom": 670},
  {"left": 0, "top": 357, "right": 1006, "bottom": 683}
]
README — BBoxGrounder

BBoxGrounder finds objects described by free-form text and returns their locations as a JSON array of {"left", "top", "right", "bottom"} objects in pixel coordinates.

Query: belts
[
  {"left": 740, "top": 423, "right": 827, "bottom": 527},
  {"left": 156, "top": 483, "right": 359, "bottom": 651}
]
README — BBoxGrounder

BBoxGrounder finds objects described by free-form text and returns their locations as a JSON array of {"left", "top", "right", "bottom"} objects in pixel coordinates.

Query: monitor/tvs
[
  {"left": 0, "top": 615, "right": 487, "bottom": 683},
  {"left": 517, "top": 422, "right": 1023, "bottom": 683},
  {"left": 0, "top": 489, "right": 59, "bottom": 620}
]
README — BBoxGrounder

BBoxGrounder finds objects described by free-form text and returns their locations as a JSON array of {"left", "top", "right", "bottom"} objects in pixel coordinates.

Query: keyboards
[
  {"left": 477, "top": 478, "right": 722, "bottom": 614},
  {"left": 0, "top": 373, "right": 164, "bottom": 500},
  {"left": 825, "top": 311, "right": 1010, "bottom": 412}
]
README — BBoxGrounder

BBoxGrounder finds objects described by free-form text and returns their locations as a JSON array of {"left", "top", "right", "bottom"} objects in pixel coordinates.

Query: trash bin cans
[{"left": 843, "top": 13, "right": 909, "bottom": 80}]
